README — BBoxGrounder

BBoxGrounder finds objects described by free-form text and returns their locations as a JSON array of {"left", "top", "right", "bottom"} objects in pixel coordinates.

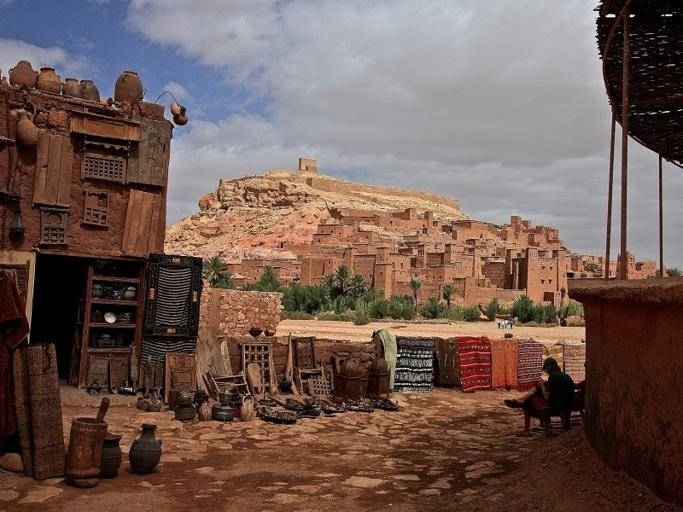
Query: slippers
[
  {"left": 515, "top": 431, "right": 534, "bottom": 437},
  {"left": 503, "top": 398, "right": 527, "bottom": 408}
]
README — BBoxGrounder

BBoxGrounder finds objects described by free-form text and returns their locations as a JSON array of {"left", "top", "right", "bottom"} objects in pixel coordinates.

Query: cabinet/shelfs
[{"left": 67, "top": 258, "right": 147, "bottom": 388}]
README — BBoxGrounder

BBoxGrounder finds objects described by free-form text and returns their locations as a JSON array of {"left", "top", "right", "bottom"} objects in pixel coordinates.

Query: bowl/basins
[{"left": 249, "top": 330, "right": 262, "bottom": 336}]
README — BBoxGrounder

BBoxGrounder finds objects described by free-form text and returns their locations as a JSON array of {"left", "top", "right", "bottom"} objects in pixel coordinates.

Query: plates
[{"left": 104, "top": 312, "right": 116, "bottom": 323}]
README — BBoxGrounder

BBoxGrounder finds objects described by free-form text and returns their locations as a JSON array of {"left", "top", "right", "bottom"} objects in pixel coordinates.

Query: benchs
[{"left": 537, "top": 378, "right": 584, "bottom": 439}]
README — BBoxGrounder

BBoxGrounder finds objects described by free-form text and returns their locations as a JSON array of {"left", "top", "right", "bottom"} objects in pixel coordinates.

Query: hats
[{"left": 539, "top": 357, "right": 557, "bottom": 372}]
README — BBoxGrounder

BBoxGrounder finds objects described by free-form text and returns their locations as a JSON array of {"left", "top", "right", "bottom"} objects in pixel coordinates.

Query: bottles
[{"left": 498, "top": 319, "right": 507, "bottom": 329}]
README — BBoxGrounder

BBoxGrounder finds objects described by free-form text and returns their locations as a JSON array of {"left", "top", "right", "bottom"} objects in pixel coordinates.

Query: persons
[{"left": 504, "top": 358, "right": 574, "bottom": 436}]
